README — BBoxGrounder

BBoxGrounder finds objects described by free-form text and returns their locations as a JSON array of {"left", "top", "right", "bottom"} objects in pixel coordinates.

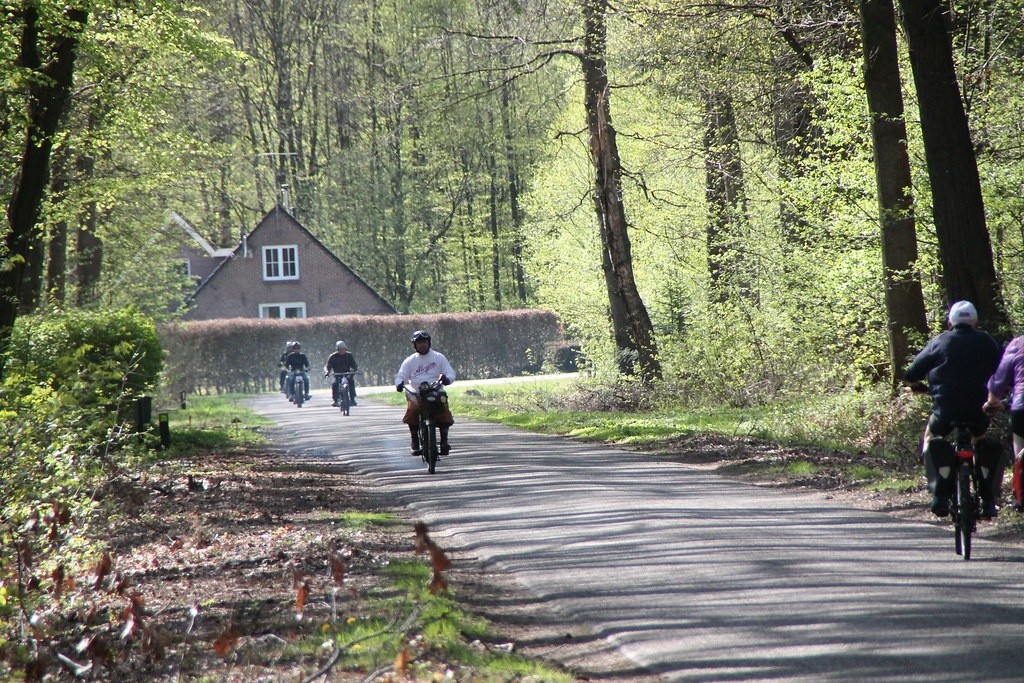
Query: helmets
[{"left": 412, "top": 331, "right": 430, "bottom": 341}]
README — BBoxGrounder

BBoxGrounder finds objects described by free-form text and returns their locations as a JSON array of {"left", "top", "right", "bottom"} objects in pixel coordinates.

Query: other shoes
[
  {"left": 410, "top": 444, "right": 420, "bottom": 455},
  {"left": 350, "top": 399, "right": 357, "bottom": 405},
  {"left": 982, "top": 504, "right": 998, "bottom": 517},
  {"left": 441, "top": 443, "right": 452, "bottom": 453},
  {"left": 331, "top": 403, "right": 339, "bottom": 406},
  {"left": 930, "top": 499, "right": 952, "bottom": 516}
]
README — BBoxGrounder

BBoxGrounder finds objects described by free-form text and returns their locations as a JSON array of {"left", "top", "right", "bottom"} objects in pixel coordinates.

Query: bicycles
[
  {"left": 911, "top": 385, "right": 992, "bottom": 559},
  {"left": 398, "top": 373, "right": 449, "bottom": 474},
  {"left": 287, "top": 367, "right": 309, "bottom": 408},
  {"left": 324, "top": 372, "right": 359, "bottom": 416},
  {"left": 983, "top": 394, "right": 1024, "bottom": 513}
]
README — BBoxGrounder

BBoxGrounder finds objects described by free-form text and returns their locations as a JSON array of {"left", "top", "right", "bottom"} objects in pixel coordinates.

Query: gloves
[
  {"left": 397, "top": 381, "right": 403, "bottom": 393},
  {"left": 441, "top": 377, "right": 449, "bottom": 385}
]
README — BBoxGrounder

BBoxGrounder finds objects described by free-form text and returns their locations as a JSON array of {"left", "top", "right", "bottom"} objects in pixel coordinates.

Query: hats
[
  {"left": 291, "top": 342, "right": 300, "bottom": 348},
  {"left": 285, "top": 342, "right": 292, "bottom": 348},
  {"left": 948, "top": 300, "right": 976, "bottom": 324},
  {"left": 336, "top": 341, "right": 346, "bottom": 349}
]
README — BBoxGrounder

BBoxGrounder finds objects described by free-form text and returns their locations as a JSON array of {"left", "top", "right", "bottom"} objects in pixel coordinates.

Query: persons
[
  {"left": 324, "top": 340, "right": 358, "bottom": 407},
  {"left": 285, "top": 341, "right": 313, "bottom": 401},
  {"left": 983, "top": 334, "right": 1024, "bottom": 458},
  {"left": 904, "top": 301, "right": 1006, "bottom": 520},
  {"left": 395, "top": 330, "right": 458, "bottom": 456},
  {"left": 279, "top": 342, "right": 295, "bottom": 393}
]
